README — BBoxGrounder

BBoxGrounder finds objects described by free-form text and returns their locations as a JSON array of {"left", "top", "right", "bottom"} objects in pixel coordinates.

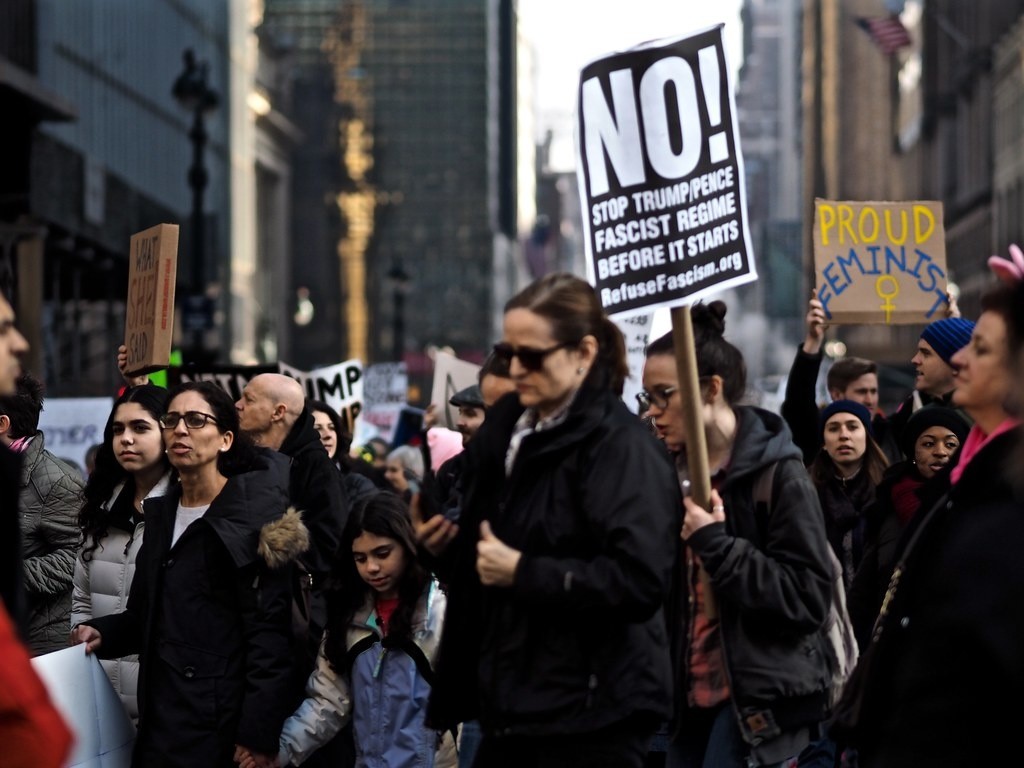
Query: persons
[
  {"left": 0, "top": 374, "right": 89, "bottom": 657},
  {"left": 0, "top": 244, "right": 1024, "bottom": 768}
]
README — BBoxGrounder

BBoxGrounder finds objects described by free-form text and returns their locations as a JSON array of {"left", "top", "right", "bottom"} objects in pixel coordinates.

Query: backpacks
[{"left": 754, "top": 454, "right": 860, "bottom": 710}]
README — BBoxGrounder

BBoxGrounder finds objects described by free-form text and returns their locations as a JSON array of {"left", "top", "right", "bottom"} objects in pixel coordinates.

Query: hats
[
  {"left": 450, "top": 384, "right": 485, "bottom": 409},
  {"left": 902, "top": 404, "right": 971, "bottom": 463},
  {"left": 818, "top": 399, "right": 872, "bottom": 436},
  {"left": 919, "top": 317, "right": 978, "bottom": 370}
]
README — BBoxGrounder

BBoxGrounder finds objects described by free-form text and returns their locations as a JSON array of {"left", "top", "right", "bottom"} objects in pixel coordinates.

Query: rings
[{"left": 713, "top": 506, "right": 724, "bottom": 510}]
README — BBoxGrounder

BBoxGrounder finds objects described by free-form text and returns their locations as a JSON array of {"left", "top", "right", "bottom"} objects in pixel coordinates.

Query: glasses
[
  {"left": 159, "top": 411, "right": 223, "bottom": 430},
  {"left": 635, "top": 377, "right": 709, "bottom": 410},
  {"left": 493, "top": 335, "right": 578, "bottom": 371}
]
